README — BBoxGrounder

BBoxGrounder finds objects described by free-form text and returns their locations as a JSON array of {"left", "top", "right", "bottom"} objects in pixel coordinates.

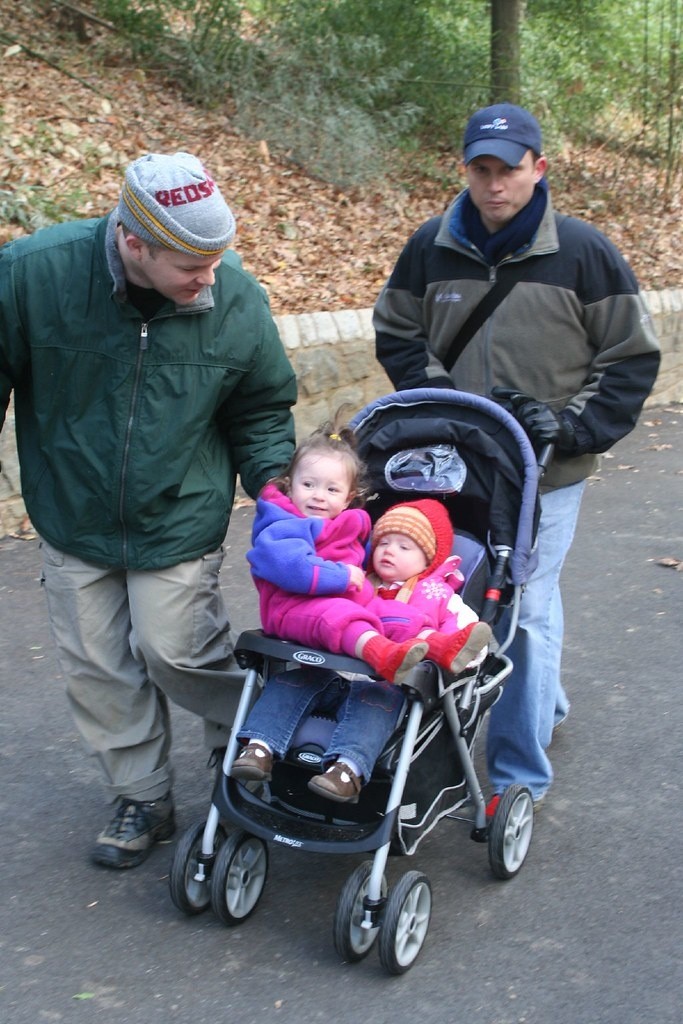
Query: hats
[
  {"left": 118, "top": 152, "right": 236, "bottom": 258},
  {"left": 462, "top": 102, "right": 541, "bottom": 166},
  {"left": 370, "top": 507, "right": 434, "bottom": 563}
]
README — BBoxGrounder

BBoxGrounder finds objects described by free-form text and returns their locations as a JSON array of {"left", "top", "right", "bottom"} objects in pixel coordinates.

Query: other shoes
[
  {"left": 232, "top": 743, "right": 273, "bottom": 781},
  {"left": 471, "top": 794, "right": 543, "bottom": 841},
  {"left": 308, "top": 761, "right": 361, "bottom": 805}
]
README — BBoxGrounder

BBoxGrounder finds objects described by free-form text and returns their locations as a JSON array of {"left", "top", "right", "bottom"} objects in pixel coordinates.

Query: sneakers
[
  {"left": 94, "top": 790, "right": 176, "bottom": 869},
  {"left": 205, "top": 746, "right": 227, "bottom": 781}
]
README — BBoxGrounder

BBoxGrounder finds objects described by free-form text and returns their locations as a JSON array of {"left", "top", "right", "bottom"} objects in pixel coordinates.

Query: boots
[
  {"left": 356, "top": 632, "right": 428, "bottom": 686},
  {"left": 416, "top": 622, "right": 492, "bottom": 675}
]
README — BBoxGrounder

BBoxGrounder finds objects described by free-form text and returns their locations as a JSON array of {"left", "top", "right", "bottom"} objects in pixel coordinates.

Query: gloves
[{"left": 493, "top": 387, "right": 576, "bottom": 449}]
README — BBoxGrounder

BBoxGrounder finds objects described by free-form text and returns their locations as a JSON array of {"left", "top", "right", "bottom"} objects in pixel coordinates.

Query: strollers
[{"left": 167, "top": 383, "right": 556, "bottom": 975}]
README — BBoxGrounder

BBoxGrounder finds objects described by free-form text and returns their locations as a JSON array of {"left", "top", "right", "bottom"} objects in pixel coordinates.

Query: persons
[
  {"left": 0, "top": 148, "right": 303, "bottom": 869},
  {"left": 376, "top": 99, "right": 663, "bottom": 821},
  {"left": 244, "top": 418, "right": 493, "bottom": 689},
  {"left": 229, "top": 495, "right": 490, "bottom": 806}
]
